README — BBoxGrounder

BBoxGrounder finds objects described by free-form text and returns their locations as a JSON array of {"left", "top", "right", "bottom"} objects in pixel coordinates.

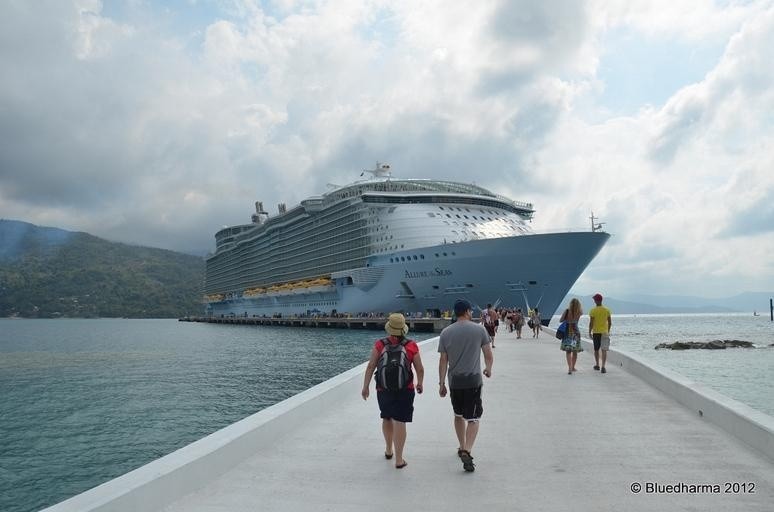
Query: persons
[
  {"left": 223, "top": 303, "right": 543, "bottom": 348},
  {"left": 360, "top": 311, "right": 424, "bottom": 470},
  {"left": 560, "top": 298, "right": 585, "bottom": 374},
  {"left": 438, "top": 301, "right": 494, "bottom": 472},
  {"left": 589, "top": 294, "right": 612, "bottom": 372}
]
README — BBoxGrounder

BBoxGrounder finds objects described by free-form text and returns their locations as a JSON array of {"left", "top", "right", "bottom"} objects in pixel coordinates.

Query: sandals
[{"left": 458, "top": 447, "right": 474, "bottom": 472}]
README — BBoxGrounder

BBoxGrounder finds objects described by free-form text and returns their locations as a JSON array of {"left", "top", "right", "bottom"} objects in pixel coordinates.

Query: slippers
[
  {"left": 396, "top": 460, "right": 407, "bottom": 468},
  {"left": 385, "top": 452, "right": 393, "bottom": 459}
]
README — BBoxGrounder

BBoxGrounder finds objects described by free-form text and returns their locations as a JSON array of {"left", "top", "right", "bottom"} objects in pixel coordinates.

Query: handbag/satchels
[
  {"left": 527, "top": 320, "right": 533, "bottom": 329},
  {"left": 556, "top": 320, "right": 569, "bottom": 340}
]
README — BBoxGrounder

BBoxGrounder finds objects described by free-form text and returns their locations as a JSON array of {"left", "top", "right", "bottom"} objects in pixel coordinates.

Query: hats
[
  {"left": 385, "top": 313, "right": 408, "bottom": 336},
  {"left": 592, "top": 294, "right": 602, "bottom": 301}
]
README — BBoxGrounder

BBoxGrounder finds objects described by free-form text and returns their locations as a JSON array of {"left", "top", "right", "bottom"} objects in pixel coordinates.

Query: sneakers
[
  {"left": 594, "top": 366, "right": 600, "bottom": 370},
  {"left": 601, "top": 368, "right": 606, "bottom": 373}
]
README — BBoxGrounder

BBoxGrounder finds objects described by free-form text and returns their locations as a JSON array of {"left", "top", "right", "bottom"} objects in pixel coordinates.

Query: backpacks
[{"left": 374, "top": 337, "right": 413, "bottom": 393}]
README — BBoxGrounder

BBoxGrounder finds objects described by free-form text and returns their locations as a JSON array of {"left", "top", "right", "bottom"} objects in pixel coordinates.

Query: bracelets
[{"left": 439, "top": 381, "right": 446, "bottom": 385}]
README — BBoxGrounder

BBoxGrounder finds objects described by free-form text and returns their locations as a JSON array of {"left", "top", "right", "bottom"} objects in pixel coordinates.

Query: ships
[{"left": 198, "top": 161, "right": 610, "bottom": 331}]
